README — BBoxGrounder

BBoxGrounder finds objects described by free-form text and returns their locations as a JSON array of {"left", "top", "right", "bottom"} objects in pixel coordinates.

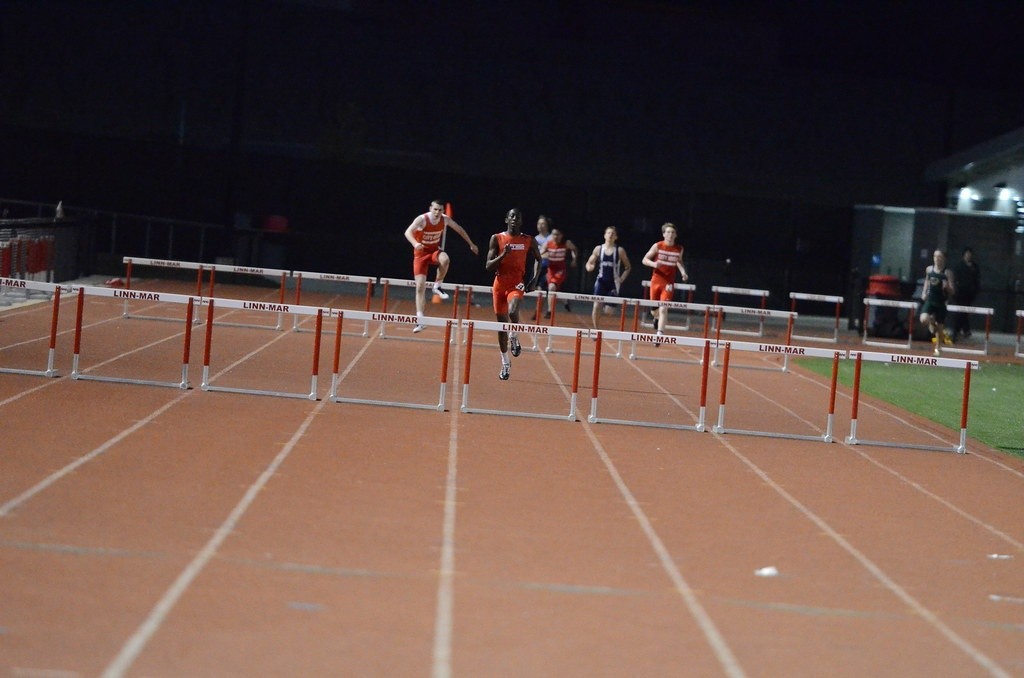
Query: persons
[
  {"left": 586, "top": 226, "right": 631, "bottom": 341},
  {"left": 404, "top": 200, "right": 478, "bottom": 333},
  {"left": 531, "top": 215, "right": 577, "bottom": 321},
  {"left": 919, "top": 248, "right": 955, "bottom": 356},
  {"left": 486, "top": 207, "right": 543, "bottom": 380},
  {"left": 954, "top": 247, "right": 981, "bottom": 335},
  {"left": 642, "top": 223, "right": 688, "bottom": 347}
]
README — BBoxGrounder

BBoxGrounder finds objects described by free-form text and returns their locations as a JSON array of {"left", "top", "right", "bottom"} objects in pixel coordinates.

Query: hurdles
[{"left": 1, "top": 235, "right": 1024, "bottom": 454}]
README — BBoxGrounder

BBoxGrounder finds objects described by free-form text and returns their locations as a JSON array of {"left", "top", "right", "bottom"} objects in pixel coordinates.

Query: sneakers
[
  {"left": 509, "top": 333, "right": 521, "bottom": 357},
  {"left": 432, "top": 286, "right": 449, "bottom": 299},
  {"left": 499, "top": 361, "right": 511, "bottom": 380},
  {"left": 413, "top": 324, "right": 423, "bottom": 333}
]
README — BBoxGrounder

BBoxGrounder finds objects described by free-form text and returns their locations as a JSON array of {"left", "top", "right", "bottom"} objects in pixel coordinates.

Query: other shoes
[
  {"left": 530, "top": 313, "right": 536, "bottom": 320},
  {"left": 934, "top": 348, "right": 943, "bottom": 357},
  {"left": 544, "top": 311, "right": 551, "bottom": 319},
  {"left": 565, "top": 304, "right": 571, "bottom": 312},
  {"left": 653, "top": 343, "right": 660, "bottom": 347},
  {"left": 653, "top": 317, "right": 659, "bottom": 330},
  {"left": 929, "top": 315, "right": 937, "bottom": 334}
]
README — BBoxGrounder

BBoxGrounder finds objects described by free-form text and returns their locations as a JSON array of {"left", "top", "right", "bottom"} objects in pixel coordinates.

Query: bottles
[{"left": 870, "top": 255, "right": 880, "bottom": 275}]
